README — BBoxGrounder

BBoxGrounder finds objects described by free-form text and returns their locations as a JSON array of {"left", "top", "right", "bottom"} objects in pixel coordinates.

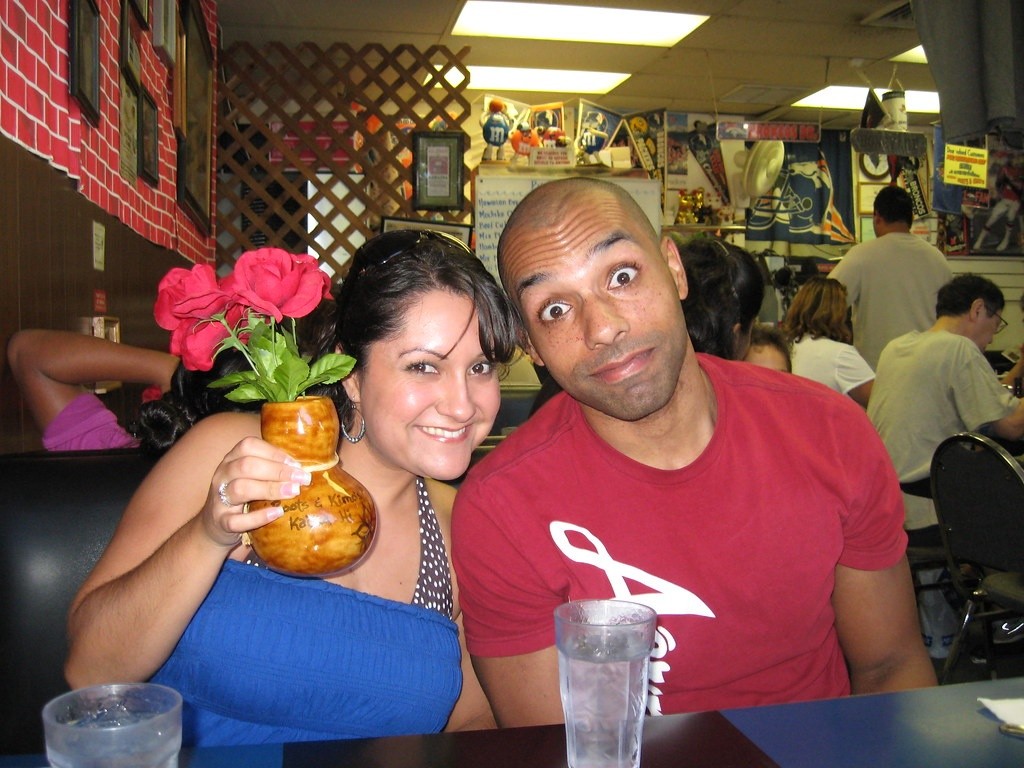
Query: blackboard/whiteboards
[{"left": 474, "top": 176, "right": 662, "bottom": 296}]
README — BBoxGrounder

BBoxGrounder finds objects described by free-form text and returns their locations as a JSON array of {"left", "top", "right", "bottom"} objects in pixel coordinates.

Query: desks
[{"left": 0, "top": 674, "right": 1024, "bottom": 768}]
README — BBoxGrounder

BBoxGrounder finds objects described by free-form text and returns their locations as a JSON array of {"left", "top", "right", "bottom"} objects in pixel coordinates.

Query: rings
[{"left": 218, "top": 480, "right": 239, "bottom": 509}]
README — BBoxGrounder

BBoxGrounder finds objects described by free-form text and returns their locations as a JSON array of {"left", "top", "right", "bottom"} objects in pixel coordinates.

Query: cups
[
  {"left": 42, "top": 681, "right": 183, "bottom": 768},
  {"left": 553, "top": 599, "right": 657, "bottom": 768}
]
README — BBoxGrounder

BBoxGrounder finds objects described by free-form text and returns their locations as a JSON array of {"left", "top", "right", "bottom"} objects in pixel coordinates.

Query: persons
[
  {"left": 64, "top": 231, "right": 532, "bottom": 746},
  {"left": 527, "top": 232, "right": 877, "bottom": 419},
  {"left": 972, "top": 150, "right": 1024, "bottom": 252},
  {"left": 868, "top": 273, "right": 1024, "bottom": 664},
  {"left": 827, "top": 186, "right": 953, "bottom": 373},
  {"left": 453, "top": 177, "right": 939, "bottom": 729},
  {"left": 7, "top": 296, "right": 353, "bottom": 451}
]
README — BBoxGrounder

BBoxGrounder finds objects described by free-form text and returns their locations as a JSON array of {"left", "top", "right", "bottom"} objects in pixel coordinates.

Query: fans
[{"left": 733, "top": 139, "right": 785, "bottom": 200}]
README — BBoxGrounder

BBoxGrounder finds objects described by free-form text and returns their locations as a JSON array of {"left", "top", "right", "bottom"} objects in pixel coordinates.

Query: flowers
[{"left": 152, "top": 247, "right": 359, "bottom": 403}]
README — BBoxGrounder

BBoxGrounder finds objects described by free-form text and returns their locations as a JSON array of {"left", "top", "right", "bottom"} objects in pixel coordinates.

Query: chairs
[
  {"left": 481, "top": 437, "right": 506, "bottom": 446},
  {"left": 473, "top": 446, "right": 496, "bottom": 465},
  {"left": 927, "top": 432, "right": 1024, "bottom": 685},
  {"left": 489, "top": 385, "right": 542, "bottom": 436},
  {"left": 0, "top": 442, "right": 176, "bottom": 757}
]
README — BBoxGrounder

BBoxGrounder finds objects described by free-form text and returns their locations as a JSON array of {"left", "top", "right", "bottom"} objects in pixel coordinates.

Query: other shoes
[{"left": 993, "top": 616, "right": 1024, "bottom": 644}]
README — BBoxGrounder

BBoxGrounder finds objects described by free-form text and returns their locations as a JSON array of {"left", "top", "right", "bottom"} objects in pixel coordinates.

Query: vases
[{"left": 246, "top": 395, "right": 378, "bottom": 581}]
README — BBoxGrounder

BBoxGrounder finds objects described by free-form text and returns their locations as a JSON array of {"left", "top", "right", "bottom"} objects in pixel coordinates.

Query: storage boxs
[{"left": 607, "top": 146, "right": 634, "bottom": 170}]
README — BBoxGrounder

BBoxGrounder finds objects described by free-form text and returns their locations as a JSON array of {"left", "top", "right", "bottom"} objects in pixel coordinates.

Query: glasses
[{"left": 985, "top": 306, "right": 1007, "bottom": 334}]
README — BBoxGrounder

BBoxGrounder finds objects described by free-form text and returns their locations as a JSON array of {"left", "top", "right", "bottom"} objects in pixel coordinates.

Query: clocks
[{"left": 859, "top": 152, "right": 890, "bottom": 180}]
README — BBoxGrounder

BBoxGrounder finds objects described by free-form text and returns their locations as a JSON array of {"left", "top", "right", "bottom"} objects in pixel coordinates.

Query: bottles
[{"left": 1012, "top": 377, "right": 1024, "bottom": 398}]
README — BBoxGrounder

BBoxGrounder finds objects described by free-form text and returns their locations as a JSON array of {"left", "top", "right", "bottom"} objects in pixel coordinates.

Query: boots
[
  {"left": 973, "top": 225, "right": 990, "bottom": 250},
  {"left": 996, "top": 223, "right": 1015, "bottom": 251}
]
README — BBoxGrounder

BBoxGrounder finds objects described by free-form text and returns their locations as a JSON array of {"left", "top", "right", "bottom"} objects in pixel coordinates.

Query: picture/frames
[
  {"left": 129, "top": 0, "right": 150, "bottom": 30},
  {"left": 177, "top": 0, "right": 213, "bottom": 239},
  {"left": 137, "top": 82, "right": 160, "bottom": 186},
  {"left": 379, "top": 214, "right": 474, "bottom": 251},
  {"left": 175, "top": 5, "right": 188, "bottom": 138},
  {"left": 120, "top": 0, "right": 144, "bottom": 95},
  {"left": 153, "top": 0, "right": 178, "bottom": 67},
  {"left": 68, "top": 0, "right": 101, "bottom": 128},
  {"left": 410, "top": 129, "right": 466, "bottom": 212},
  {"left": 857, "top": 181, "right": 890, "bottom": 216}
]
